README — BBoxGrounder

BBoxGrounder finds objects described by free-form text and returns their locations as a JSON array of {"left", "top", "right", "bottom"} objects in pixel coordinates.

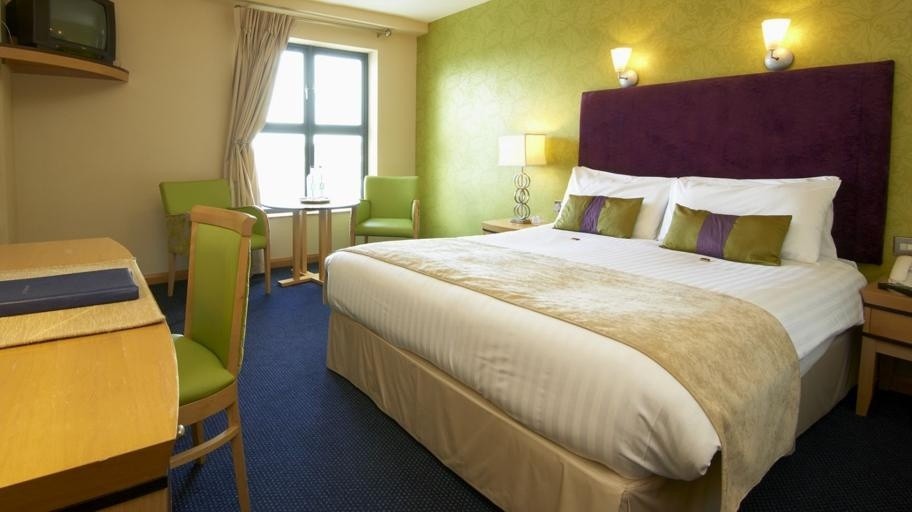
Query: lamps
[
  {"left": 496, "top": 133, "right": 560, "bottom": 225},
  {"left": 760, "top": 18, "right": 796, "bottom": 69},
  {"left": 609, "top": 47, "right": 640, "bottom": 87}
]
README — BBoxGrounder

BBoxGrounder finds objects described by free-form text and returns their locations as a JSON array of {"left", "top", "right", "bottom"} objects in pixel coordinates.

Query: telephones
[{"left": 888, "top": 256, "right": 912, "bottom": 291}]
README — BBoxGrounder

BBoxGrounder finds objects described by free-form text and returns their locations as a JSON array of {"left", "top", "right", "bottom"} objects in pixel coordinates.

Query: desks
[
  {"left": 0, "top": 236, "right": 178, "bottom": 511},
  {"left": 265, "top": 198, "right": 361, "bottom": 288}
]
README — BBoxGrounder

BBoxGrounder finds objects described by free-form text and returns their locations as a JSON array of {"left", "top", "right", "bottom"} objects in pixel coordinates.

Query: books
[{"left": 0, "top": 265, "right": 141, "bottom": 318}]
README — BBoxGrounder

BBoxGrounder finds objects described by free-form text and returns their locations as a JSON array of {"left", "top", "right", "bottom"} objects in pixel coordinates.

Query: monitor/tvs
[{"left": 6, "top": 0, "right": 115, "bottom": 66}]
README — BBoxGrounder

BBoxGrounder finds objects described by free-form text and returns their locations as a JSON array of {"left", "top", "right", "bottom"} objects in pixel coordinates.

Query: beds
[{"left": 322, "top": 58, "right": 889, "bottom": 510}]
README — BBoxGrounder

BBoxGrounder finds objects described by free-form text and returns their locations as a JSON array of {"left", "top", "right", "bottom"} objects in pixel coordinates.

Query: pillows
[
  {"left": 818, "top": 202, "right": 838, "bottom": 262},
  {"left": 556, "top": 192, "right": 644, "bottom": 240},
  {"left": 656, "top": 172, "right": 844, "bottom": 260},
  {"left": 554, "top": 166, "right": 673, "bottom": 242},
  {"left": 659, "top": 201, "right": 793, "bottom": 269}
]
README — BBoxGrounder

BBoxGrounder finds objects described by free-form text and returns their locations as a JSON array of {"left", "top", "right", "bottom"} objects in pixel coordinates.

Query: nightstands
[
  {"left": 481, "top": 218, "right": 550, "bottom": 237},
  {"left": 854, "top": 272, "right": 911, "bottom": 418}
]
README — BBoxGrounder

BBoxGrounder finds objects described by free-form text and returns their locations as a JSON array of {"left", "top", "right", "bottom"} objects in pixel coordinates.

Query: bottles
[{"left": 554, "top": 200, "right": 561, "bottom": 215}]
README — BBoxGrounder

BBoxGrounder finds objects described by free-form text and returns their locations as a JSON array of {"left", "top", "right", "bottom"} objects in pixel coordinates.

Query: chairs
[
  {"left": 158, "top": 179, "right": 274, "bottom": 298},
  {"left": 350, "top": 174, "right": 422, "bottom": 246},
  {"left": 154, "top": 204, "right": 258, "bottom": 510}
]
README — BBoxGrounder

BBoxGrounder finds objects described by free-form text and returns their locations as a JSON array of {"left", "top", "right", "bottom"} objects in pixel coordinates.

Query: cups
[{"left": 530, "top": 215, "right": 540, "bottom": 225}]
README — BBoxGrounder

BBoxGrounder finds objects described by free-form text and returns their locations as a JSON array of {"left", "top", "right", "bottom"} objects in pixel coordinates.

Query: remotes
[{"left": 878, "top": 281, "right": 912, "bottom": 299}]
